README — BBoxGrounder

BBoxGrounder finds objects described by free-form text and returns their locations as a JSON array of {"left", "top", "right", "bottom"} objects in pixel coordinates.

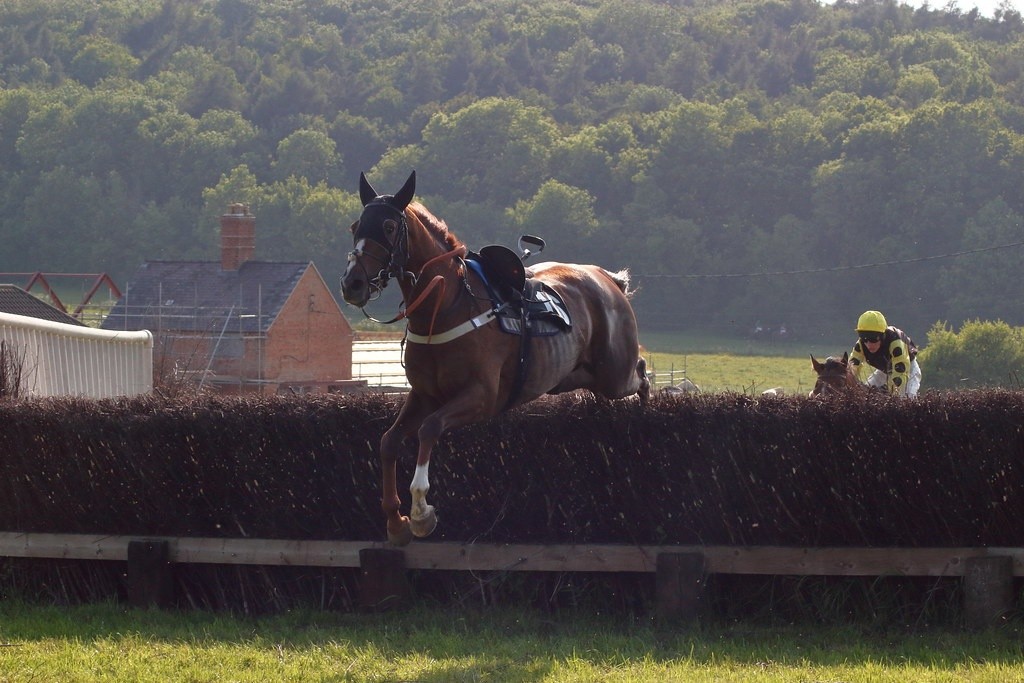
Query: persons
[{"left": 849, "top": 311, "right": 922, "bottom": 399}]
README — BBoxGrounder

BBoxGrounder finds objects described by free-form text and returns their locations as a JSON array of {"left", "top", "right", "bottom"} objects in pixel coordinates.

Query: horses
[
  {"left": 808, "top": 352, "right": 863, "bottom": 398},
  {"left": 337, "top": 167, "right": 653, "bottom": 550}
]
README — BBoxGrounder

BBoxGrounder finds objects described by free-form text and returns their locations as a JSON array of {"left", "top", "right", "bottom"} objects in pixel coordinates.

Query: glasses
[{"left": 860, "top": 335, "right": 883, "bottom": 343}]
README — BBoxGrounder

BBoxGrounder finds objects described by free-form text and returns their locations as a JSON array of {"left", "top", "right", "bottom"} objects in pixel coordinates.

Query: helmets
[{"left": 854, "top": 311, "right": 887, "bottom": 333}]
